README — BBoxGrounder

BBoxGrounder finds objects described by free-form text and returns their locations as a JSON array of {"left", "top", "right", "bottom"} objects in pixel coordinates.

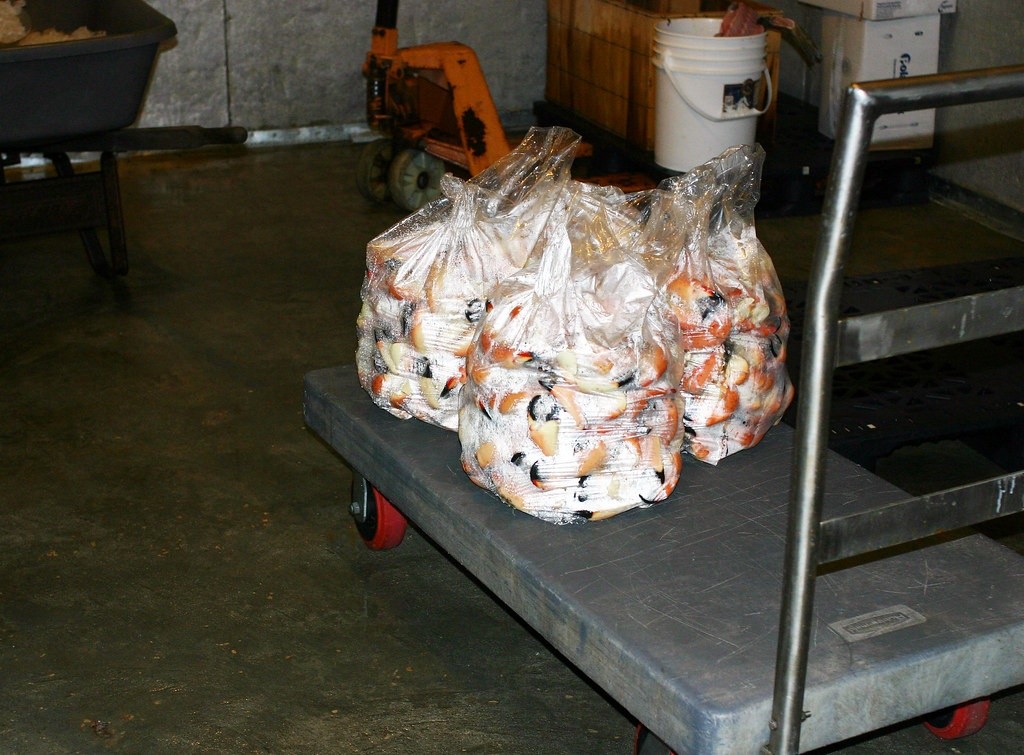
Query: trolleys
[{"left": 305, "top": 63, "right": 1023, "bottom": 755}]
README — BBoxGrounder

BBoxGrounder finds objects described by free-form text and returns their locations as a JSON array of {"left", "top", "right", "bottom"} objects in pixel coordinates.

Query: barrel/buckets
[{"left": 650, "top": 17, "right": 773, "bottom": 172}]
